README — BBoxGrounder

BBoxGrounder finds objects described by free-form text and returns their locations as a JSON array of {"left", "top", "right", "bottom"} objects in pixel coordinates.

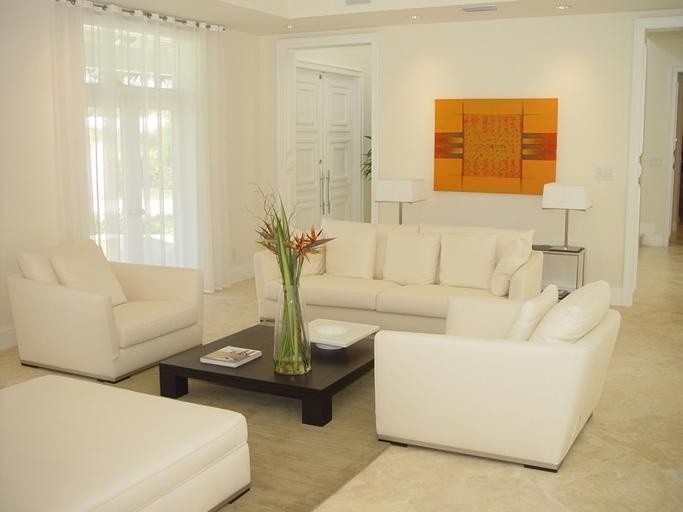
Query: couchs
[{"left": 252, "top": 218, "right": 543, "bottom": 335}]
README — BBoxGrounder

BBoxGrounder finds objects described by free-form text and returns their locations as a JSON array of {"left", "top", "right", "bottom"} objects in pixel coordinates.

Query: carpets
[{"left": 108, "top": 366, "right": 387, "bottom": 511}]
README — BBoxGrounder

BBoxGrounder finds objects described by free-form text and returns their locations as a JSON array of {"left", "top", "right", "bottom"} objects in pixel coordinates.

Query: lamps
[
  {"left": 373, "top": 179, "right": 429, "bottom": 224},
  {"left": 542, "top": 181, "right": 593, "bottom": 252}
]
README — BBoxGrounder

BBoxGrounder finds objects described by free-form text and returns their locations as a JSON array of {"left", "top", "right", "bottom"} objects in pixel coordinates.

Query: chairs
[
  {"left": 5, "top": 238, "right": 204, "bottom": 384},
  {"left": 374, "top": 290, "right": 621, "bottom": 473}
]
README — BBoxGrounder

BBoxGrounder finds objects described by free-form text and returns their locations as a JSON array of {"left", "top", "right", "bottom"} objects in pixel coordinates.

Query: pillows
[
  {"left": 489, "top": 229, "right": 536, "bottom": 297},
  {"left": 15, "top": 238, "right": 97, "bottom": 287},
  {"left": 502, "top": 282, "right": 559, "bottom": 342},
  {"left": 50, "top": 246, "right": 128, "bottom": 309},
  {"left": 322, "top": 217, "right": 379, "bottom": 282},
  {"left": 527, "top": 280, "right": 612, "bottom": 346},
  {"left": 439, "top": 234, "right": 498, "bottom": 291},
  {"left": 379, "top": 226, "right": 442, "bottom": 286}
]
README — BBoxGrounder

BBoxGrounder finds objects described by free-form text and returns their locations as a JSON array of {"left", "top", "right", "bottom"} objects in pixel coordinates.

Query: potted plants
[{"left": 256, "top": 187, "right": 337, "bottom": 376}]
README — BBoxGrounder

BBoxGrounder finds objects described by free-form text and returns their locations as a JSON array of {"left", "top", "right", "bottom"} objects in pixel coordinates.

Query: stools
[{"left": 0, "top": 373, "right": 251, "bottom": 511}]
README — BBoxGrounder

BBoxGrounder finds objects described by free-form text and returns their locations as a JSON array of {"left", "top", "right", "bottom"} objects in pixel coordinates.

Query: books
[{"left": 199, "top": 344, "right": 262, "bottom": 369}]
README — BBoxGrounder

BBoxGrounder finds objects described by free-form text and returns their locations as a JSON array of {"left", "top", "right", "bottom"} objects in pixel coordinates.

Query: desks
[{"left": 531, "top": 245, "right": 586, "bottom": 301}]
